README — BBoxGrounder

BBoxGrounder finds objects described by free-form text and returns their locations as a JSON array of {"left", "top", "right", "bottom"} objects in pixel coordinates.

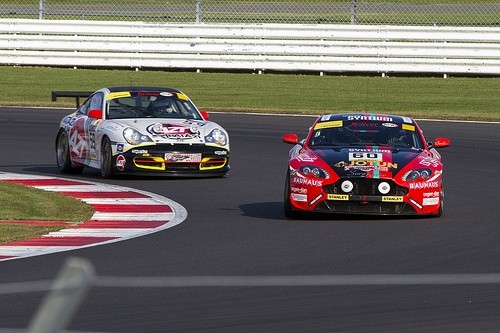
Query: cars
[
  {"left": 282, "top": 112, "right": 450, "bottom": 219},
  {"left": 51, "top": 86, "right": 231, "bottom": 180}
]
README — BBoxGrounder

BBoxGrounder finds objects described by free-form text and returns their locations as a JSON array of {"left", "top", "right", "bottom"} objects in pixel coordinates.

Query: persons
[
  {"left": 383, "top": 126, "right": 407, "bottom": 144},
  {"left": 150, "top": 98, "right": 174, "bottom": 115}
]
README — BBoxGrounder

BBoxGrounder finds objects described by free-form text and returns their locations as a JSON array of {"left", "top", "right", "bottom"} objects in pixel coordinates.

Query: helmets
[{"left": 151, "top": 97, "right": 173, "bottom": 115}]
